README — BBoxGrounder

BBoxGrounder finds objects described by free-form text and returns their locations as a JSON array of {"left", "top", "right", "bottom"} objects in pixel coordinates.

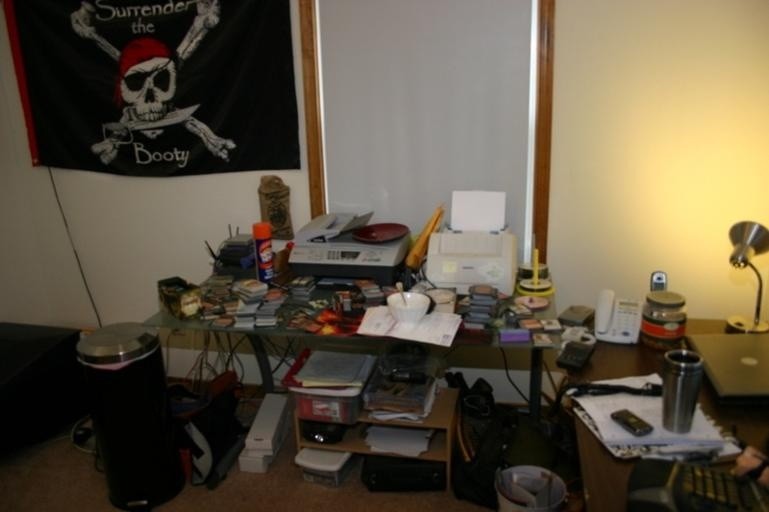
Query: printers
[{"left": 426, "top": 222, "right": 519, "bottom": 298}]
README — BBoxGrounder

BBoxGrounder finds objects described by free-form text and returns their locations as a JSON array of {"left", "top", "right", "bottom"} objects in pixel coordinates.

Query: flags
[{"left": 0, "top": 0, "right": 305, "bottom": 178}]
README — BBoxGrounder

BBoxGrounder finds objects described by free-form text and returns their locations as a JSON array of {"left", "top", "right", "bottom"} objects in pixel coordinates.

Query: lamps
[{"left": 721, "top": 218, "right": 769, "bottom": 334}]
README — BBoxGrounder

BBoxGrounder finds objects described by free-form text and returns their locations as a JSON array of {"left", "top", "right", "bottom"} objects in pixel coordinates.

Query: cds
[{"left": 513, "top": 262, "right": 552, "bottom": 311}]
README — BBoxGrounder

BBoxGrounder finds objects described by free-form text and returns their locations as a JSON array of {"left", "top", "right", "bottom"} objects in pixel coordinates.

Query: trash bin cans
[
  {"left": 75, "top": 323, "right": 184, "bottom": 512},
  {"left": 496, "top": 465, "right": 566, "bottom": 512}
]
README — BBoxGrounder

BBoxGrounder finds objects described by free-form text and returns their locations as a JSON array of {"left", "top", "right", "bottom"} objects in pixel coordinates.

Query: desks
[
  {"left": 140, "top": 273, "right": 564, "bottom": 494},
  {"left": 574, "top": 314, "right": 769, "bottom": 511}
]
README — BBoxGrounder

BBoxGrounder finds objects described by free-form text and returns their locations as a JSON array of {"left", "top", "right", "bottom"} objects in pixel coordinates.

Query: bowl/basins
[{"left": 384, "top": 292, "right": 431, "bottom": 327}]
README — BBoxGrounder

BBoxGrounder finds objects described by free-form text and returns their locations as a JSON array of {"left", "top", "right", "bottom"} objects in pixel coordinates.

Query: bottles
[{"left": 252, "top": 222, "right": 275, "bottom": 283}]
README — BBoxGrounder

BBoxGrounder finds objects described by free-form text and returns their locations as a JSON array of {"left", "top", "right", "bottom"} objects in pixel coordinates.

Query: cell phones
[
  {"left": 650, "top": 270, "right": 667, "bottom": 290},
  {"left": 611, "top": 409, "right": 652, "bottom": 436}
]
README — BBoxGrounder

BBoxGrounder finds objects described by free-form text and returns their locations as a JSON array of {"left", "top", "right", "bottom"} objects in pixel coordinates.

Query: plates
[{"left": 352, "top": 222, "right": 409, "bottom": 243}]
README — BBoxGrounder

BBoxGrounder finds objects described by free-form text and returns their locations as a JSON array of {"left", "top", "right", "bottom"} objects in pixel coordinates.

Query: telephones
[{"left": 594, "top": 289, "right": 644, "bottom": 345}]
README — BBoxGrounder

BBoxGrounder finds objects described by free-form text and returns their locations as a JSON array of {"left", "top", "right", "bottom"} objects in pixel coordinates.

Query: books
[
  {"left": 565, "top": 373, "right": 721, "bottom": 447},
  {"left": 361, "top": 359, "right": 439, "bottom": 417}
]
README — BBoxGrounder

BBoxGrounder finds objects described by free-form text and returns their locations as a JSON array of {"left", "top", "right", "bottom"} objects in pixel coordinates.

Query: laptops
[
  {"left": 624, "top": 457, "right": 769, "bottom": 512},
  {"left": 685, "top": 333, "right": 769, "bottom": 405}
]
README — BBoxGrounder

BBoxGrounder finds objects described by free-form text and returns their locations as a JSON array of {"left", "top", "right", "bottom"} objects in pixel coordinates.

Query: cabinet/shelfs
[{"left": 292, "top": 386, "right": 460, "bottom": 491}]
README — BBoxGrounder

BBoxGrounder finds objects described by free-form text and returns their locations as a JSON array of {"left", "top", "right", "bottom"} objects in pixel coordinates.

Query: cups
[{"left": 660, "top": 347, "right": 705, "bottom": 435}]
